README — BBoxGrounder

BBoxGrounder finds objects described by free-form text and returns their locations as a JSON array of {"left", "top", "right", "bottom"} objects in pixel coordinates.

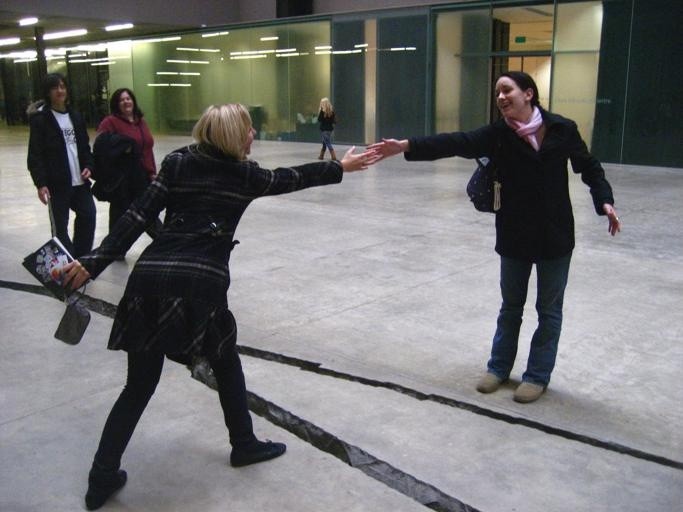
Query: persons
[
  {"left": 91, "top": 88, "right": 165, "bottom": 260},
  {"left": 23, "top": 72, "right": 97, "bottom": 257},
  {"left": 316, "top": 97, "right": 337, "bottom": 161},
  {"left": 364, "top": 69, "right": 622, "bottom": 405},
  {"left": 59, "top": 102, "right": 379, "bottom": 512}
]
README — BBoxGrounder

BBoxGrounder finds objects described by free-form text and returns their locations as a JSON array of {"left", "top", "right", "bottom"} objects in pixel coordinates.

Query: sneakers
[
  {"left": 514, "top": 380, "right": 548, "bottom": 403},
  {"left": 84, "top": 468, "right": 126, "bottom": 510},
  {"left": 230, "top": 440, "right": 286, "bottom": 467},
  {"left": 475, "top": 372, "right": 509, "bottom": 393}
]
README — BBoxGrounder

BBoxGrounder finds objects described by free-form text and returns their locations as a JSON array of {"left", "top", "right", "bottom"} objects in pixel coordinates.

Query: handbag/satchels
[
  {"left": 54, "top": 302, "right": 91, "bottom": 345},
  {"left": 466, "top": 157, "right": 499, "bottom": 213}
]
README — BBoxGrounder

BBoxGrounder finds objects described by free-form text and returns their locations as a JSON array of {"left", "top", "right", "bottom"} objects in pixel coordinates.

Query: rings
[{"left": 614, "top": 216, "right": 619, "bottom": 223}]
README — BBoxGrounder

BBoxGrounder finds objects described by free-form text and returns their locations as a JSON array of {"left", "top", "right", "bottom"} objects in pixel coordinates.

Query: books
[{"left": 22, "top": 234, "right": 91, "bottom": 303}]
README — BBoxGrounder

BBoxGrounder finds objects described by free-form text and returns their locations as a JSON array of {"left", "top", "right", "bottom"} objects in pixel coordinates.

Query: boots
[
  {"left": 318, "top": 150, "right": 325, "bottom": 160},
  {"left": 330, "top": 149, "right": 336, "bottom": 160}
]
